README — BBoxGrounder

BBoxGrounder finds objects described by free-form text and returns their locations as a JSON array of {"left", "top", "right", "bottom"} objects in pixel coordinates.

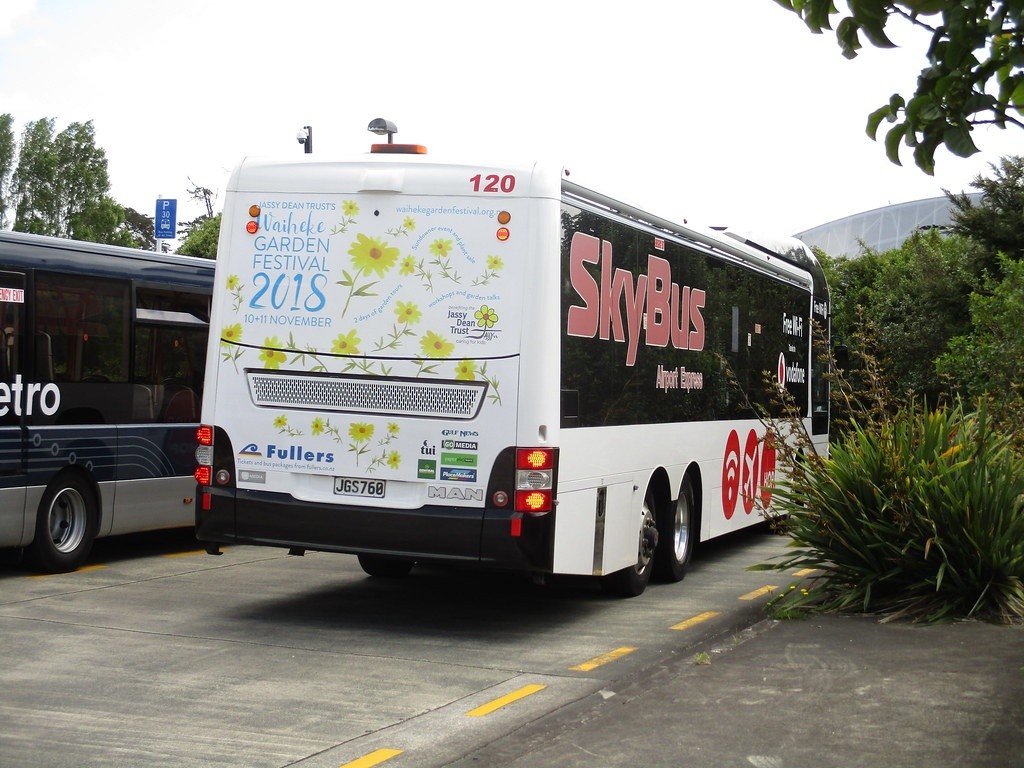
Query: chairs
[{"left": 34, "top": 331, "right": 198, "bottom": 426}]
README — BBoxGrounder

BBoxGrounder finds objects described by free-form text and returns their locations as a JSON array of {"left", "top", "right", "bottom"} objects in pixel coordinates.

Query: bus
[
  {"left": 0, "top": 228, "right": 216, "bottom": 574},
  {"left": 190, "top": 118, "right": 850, "bottom": 599}
]
